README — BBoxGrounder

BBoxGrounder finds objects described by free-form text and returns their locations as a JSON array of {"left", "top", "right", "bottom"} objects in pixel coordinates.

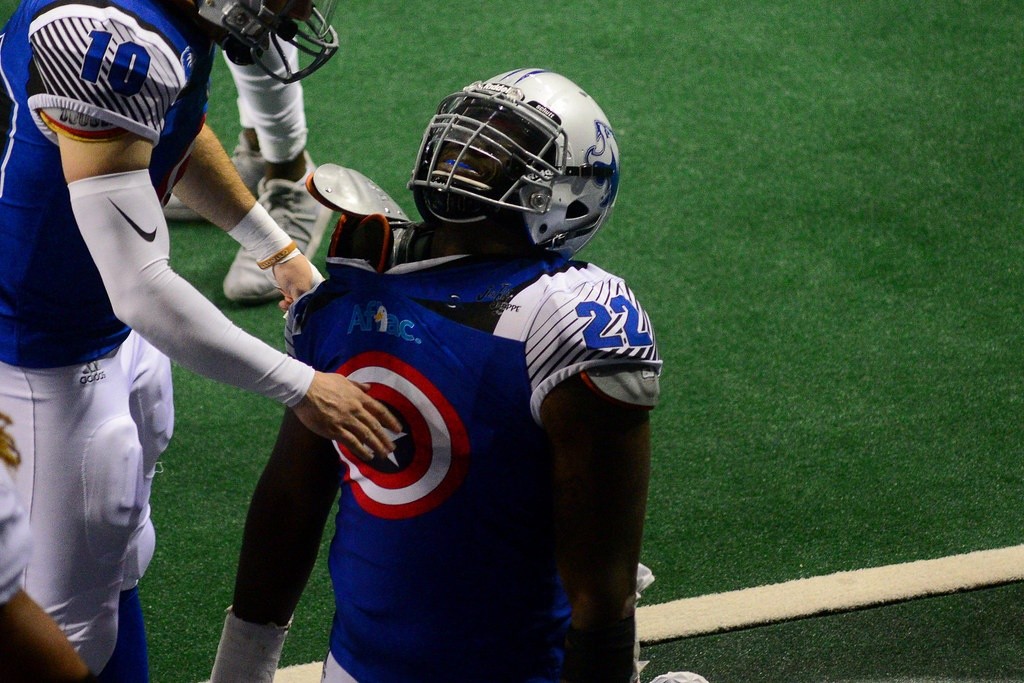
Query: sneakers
[
  {"left": 161, "top": 131, "right": 265, "bottom": 220},
  {"left": 223, "top": 151, "right": 332, "bottom": 304}
]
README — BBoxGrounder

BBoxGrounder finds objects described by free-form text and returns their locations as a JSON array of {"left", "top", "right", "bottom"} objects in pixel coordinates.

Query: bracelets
[{"left": 227, "top": 198, "right": 304, "bottom": 273}]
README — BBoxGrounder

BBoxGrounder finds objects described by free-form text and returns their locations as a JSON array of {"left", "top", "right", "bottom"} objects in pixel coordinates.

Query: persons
[
  {"left": 0, "top": 0, "right": 402, "bottom": 683},
  {"left": 162, "top": 0, "right": 332, "bottom": 303},
  {"left": 208, "top": 65, "right": 708, "bottom": 683},
  {"left": 0, "top": 413, "right": 96, "bottom": 683}
]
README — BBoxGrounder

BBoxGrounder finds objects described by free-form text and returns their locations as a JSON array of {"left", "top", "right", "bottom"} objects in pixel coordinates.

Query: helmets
[
  {"left": 182, "top": 0, "right": 337, "bottom": 82},
  {"left": 407, "top": 68, "right": 623, "bottom": 261}
]
